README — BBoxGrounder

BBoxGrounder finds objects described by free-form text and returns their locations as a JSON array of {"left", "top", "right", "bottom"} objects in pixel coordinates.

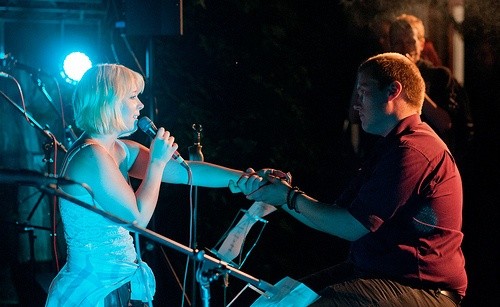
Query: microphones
[
  {"left": 136, "top": 117, "right": 190, "bottom": 171},
  {"left": 0, "top": 167, "right": 76, "bottom": 186},
  {"left": 3, "top": 58, "right": 54, "bottom": 78}
]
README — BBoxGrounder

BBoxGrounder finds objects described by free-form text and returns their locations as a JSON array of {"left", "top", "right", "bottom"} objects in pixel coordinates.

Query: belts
[{"left": 438, "top": 285, "right": 461, "bottom": 304}]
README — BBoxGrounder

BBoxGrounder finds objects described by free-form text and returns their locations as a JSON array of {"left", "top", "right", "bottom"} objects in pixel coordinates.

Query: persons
[
  {"left": 227, "top": 52, "right": 467, "bottom": 307},
  {"left": 363, "top": 17, "right": 442, "bottom": 67},
  {"left": 43, "top": 64, "right": 292, "bottom": 307},
  {"left": 348, "top": 14, "right": 468, "bottom": 236}
]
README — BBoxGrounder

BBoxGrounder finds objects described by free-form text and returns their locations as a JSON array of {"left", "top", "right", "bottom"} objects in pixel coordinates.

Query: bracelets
[{"left": 285, "top": 186, "right": 304, "bottom": 213}]
